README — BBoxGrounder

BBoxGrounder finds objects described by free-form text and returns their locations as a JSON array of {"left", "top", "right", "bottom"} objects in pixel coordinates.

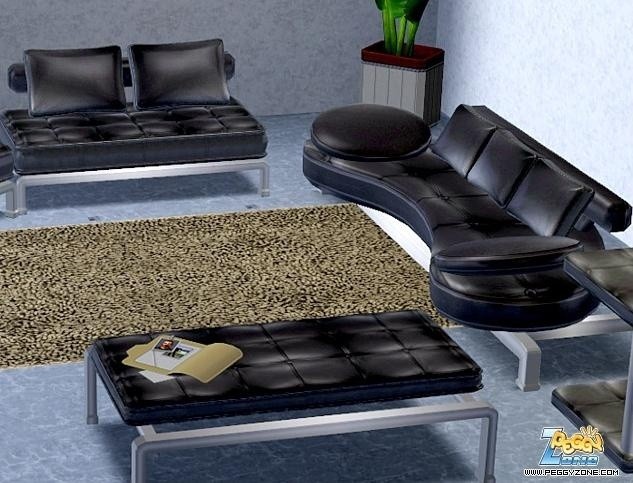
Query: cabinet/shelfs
[{"left": 550, "top": 243, "right": 632, "bottom": 475}]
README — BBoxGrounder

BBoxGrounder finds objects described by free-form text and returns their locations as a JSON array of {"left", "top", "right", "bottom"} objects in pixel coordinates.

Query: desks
[{"left": 84, "top": 309, "right": 496, "bottom": 482}]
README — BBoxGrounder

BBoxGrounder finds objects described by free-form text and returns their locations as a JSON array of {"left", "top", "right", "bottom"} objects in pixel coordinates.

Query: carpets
[{"left": 1, "top": 200, "right": 466, "bottom": 365}]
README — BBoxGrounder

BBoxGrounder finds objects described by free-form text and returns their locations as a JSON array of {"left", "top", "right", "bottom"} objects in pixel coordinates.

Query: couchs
[
  {"left": 1, "top": 36, "right": 274, "bottom": 216},
  {"left": 299, "top": 102, "right": 633, "bottom": 394}
]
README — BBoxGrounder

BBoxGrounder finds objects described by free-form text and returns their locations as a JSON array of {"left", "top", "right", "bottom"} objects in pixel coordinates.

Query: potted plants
[{"left": 360, "top": 0, "right": 445, "bottom": 128}]
